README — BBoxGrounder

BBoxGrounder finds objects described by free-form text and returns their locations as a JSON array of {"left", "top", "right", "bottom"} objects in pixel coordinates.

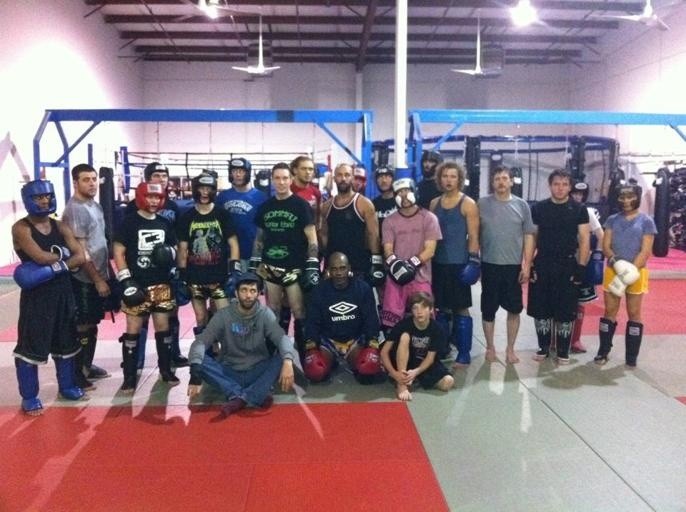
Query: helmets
[
  {"left": 228, "top": 158, "right": 251, "bottom": 183},
  {"left": 144, "top": 162, "right": 169, "bottom": 181},
  {"left": 374, "top": 165, "right": 394, "bottom": 192},
  {"left": 136, "top": 182, "right": 166, "bottom": 210},
  {"left": 615, "top": 178, "right": 642, "bottom": 209},
  {"left": 192, "top": 173, "right": 217, "bottom": 203},
  {"left": 569, "top": 179, "right": 589, "bottom": 202},
  {"left": 21, "top": 180, "right": 56, "bottom": 217},
  {"left": 352, "top": 167, "right": 367, "bottom": 191},
  {"left": 421, "top": 149, "right": 442, "bottom": 177},
  {"left": 391, "top": 177, "right": 418, "bottom": 209}
]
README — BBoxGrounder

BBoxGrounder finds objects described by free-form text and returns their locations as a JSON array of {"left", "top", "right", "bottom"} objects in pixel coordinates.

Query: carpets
[
  {"left": 516, "top": 274, "right": 686, "bottom": 341},
  {"left": 602, "top": 244, "right": 686, "bottom": 274},
  {"left": 0, "top": 394, "right": 454, "bottom": 512},
  {"left": 0, "top": 261, "right": 210, "bottom": 344}
]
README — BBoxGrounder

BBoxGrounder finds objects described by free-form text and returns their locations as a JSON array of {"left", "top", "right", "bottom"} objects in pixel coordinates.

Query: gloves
[
  {"left": 153, "top": 243, "right": 177, "bottom": 268},
  {"left": 385, "top": 254, "right": 423, "bottom": 286},
  {"left": 117, "top": 268, "right": 146, "bottom": 307},
  {"left": 305, "top": 348, "right": 329, "bottom": 382},
  {"left": 171, "top": 268, "right": 191, "bottom": 305},
  {"left": 299, "top": 257, "right": 321, "bottom": 291},
  {"left": 607, "top": 255, "right": 640, "bottom": 297},
  {"left": 366, "top": 253, "right": 386, "bottom": 286},
  {"left": 223, "top": 259, "right": 243, "bottom": 297},
  {"left": 458, "top": 253, "right": 481, "bottom": 285},
  {"left": 248, "top": 256, "right": 264, "bottom": 290},
  {"left": 13, "top": 259, "right": 69, "bottom": 290},
  {"left": 355, "top": 348, "right": 381, "bottom": 375},
  {"left": 584, "top": 250, "right": 603, "bottom": 285},
  {"left": 51, "top": 244, "right": 80, "bottom": 273}
]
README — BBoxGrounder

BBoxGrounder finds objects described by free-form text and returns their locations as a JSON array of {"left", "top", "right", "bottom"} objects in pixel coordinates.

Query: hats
[{"left": 236, "top": 272, "right": 260, "bottom": 286}]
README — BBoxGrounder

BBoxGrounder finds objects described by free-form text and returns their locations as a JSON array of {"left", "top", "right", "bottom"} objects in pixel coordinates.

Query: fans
[
  {"left": 230, "top": 16, "right": 281, "bottom": 76},
  {"left": 171, "top": 0, "right": 263, "bottom": 25},
  {"left": 584, "top": 0, "right": 671, "bottom": 31},
  {"left": 447, "top": 8, "right": 502, "bottom": 82}
]
